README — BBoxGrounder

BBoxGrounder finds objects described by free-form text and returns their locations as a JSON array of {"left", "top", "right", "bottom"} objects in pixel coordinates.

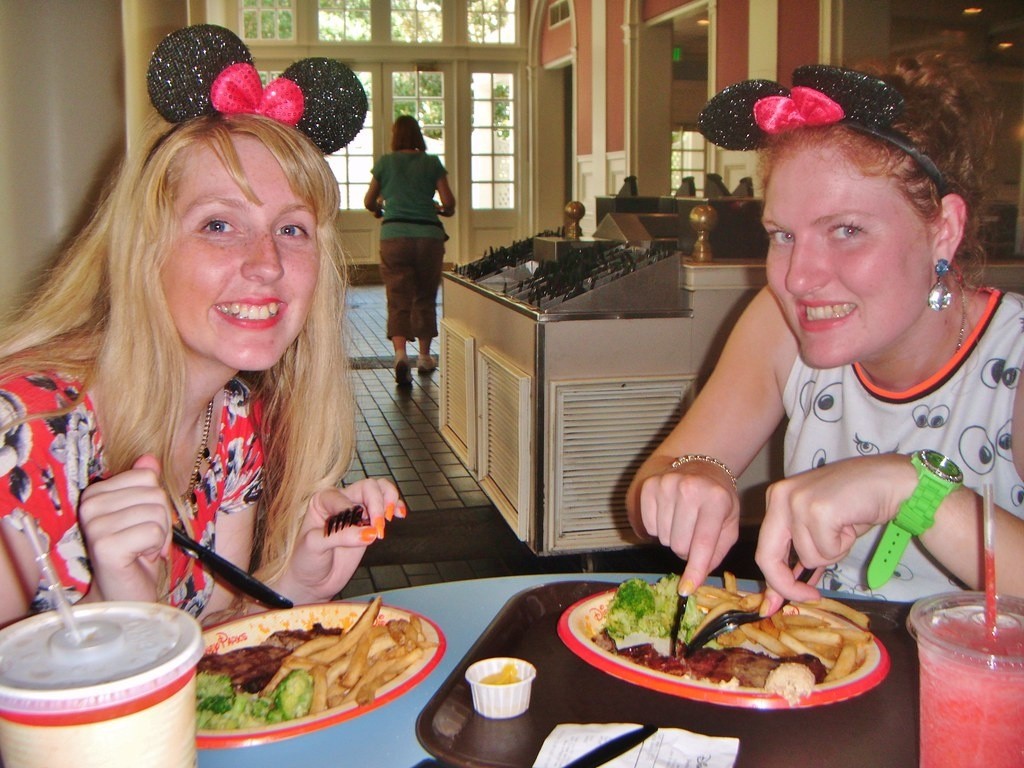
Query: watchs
[{"left": 864, "top": 449, "right": 964, "bottom": 589}]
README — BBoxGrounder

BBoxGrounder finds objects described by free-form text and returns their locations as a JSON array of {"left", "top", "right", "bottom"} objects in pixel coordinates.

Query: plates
[
  {"left": 557, "top": 586, "right": 889, "bottom": 712},
  {"left": 194, "top": 602, "right": 448, "bottom": 750}
]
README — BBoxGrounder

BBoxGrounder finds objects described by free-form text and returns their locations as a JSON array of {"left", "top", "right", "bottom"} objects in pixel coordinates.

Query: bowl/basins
[{"left": 464, "top": 656, "right": 537, "bottom": 718}]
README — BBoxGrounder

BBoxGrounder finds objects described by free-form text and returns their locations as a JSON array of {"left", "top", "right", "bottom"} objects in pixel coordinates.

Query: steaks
[
  {"left": 196, "top": 622, "right": 343, "bottom": 694},
  {"left": 591, "top": 630, "right": 828, "bottom": 688}
]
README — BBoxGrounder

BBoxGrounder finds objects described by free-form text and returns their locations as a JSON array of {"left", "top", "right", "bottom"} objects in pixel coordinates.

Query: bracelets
[{"left": 666, "top": 454, "right": 738, "bottom": 492}]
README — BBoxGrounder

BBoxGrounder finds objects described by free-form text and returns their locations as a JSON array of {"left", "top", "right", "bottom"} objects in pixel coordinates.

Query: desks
[{"left": 196, "top": 573, "right": 883, "bottom": 768}]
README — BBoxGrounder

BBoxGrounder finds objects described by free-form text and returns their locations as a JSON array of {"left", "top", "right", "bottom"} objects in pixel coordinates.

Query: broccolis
[
  {"left": 194, "top": 669, "right": 314, "bottom": 730},
  {"left": 606, "top": 574, "right": 706, "bottom": 639}
]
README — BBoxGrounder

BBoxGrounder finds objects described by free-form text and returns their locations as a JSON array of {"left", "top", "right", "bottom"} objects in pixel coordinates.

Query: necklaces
[
  {"left": 179, "top": 395, "right": 215, "bottom": 504},
  {"left": 955, "top": 278, "right": 966, "bottom": 355}
]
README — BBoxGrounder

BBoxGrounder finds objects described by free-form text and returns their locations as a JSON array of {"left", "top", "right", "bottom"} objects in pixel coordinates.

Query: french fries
[
  {"left": 261, "top": 593, "right": 439, "bottom": 715},
  {"left": 692, "top": 571, "right": 873, "bottom": 682}
]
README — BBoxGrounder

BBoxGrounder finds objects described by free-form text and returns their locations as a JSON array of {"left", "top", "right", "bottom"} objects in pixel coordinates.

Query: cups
[
  {"left": 905, "top": 590, "right": 1024, "bottom": 768},
  {"left": 0, "top": 602, "right": 204, "bottom": 768}
]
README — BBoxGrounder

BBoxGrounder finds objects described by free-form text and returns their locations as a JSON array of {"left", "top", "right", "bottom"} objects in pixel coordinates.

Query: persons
[
  {"left": 1, "top": 114, "right": 411, "bottom": 637},
  {"left": 364, "top": 114, "right": 457, "bottom": 388},
  {"left": 617, "top": 50, "right": 1024, "bottom": 603}
]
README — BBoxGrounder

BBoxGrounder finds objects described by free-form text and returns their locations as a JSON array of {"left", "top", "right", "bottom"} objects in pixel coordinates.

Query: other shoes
[
  {"left": 416, "top": 357, "right": 436, "bottom": 373},
  {"left": 394, "top": 356, "right": 413, "bottom": 384}
]
